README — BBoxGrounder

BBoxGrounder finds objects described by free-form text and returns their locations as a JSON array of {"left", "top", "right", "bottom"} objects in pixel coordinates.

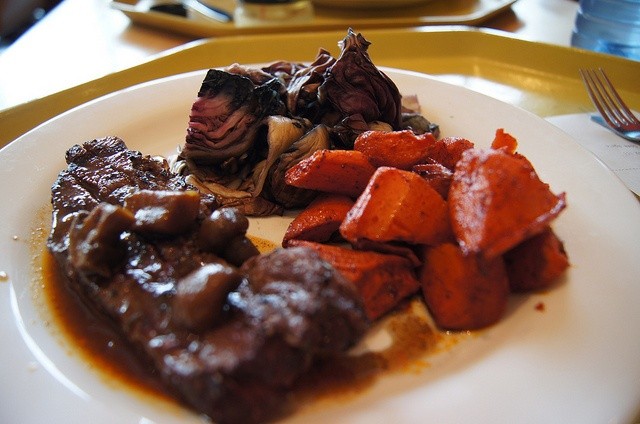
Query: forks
[{"left": 578, "top": 66, "right": 640, "bottom": 145}]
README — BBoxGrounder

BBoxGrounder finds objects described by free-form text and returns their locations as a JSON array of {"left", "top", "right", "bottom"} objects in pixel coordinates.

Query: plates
[{"left": 109, "top": 1, "right": 518, "bottom": 37}]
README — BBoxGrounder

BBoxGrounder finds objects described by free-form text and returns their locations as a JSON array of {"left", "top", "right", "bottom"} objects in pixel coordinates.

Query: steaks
[{"left": 43, "top": 135, "right": 366, "bottom": 424}]
